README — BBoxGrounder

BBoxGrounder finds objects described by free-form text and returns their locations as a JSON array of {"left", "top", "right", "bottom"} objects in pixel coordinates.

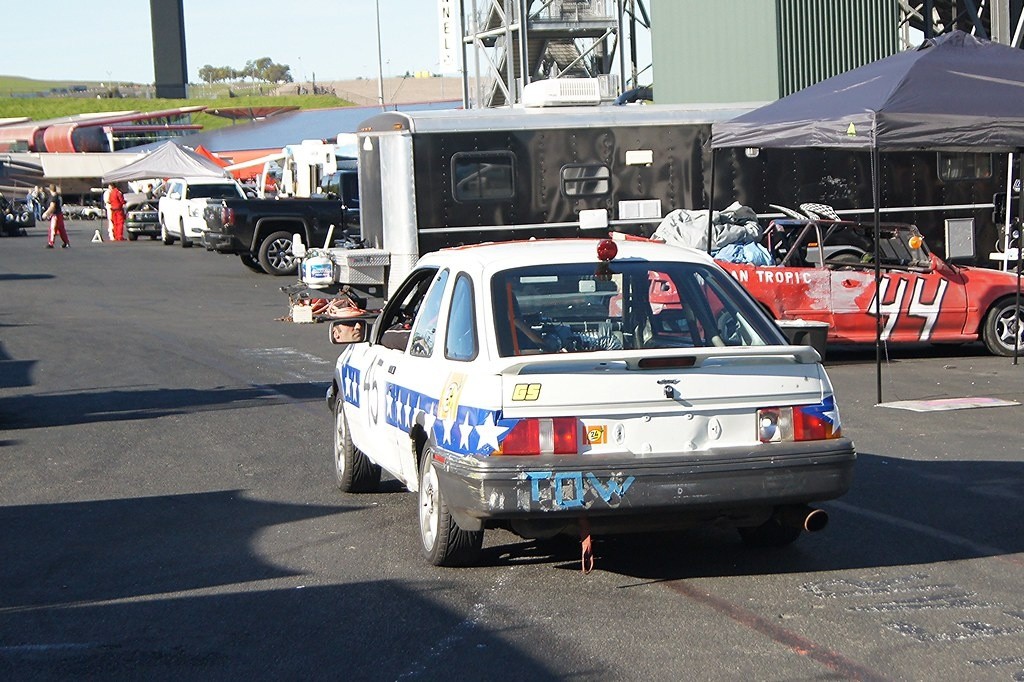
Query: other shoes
[
  {"left": 46, "top": 245, "right": 55, "bottom": 249},
  {"left": 60, "top": 242, "right": 70, "bottom": 249}
]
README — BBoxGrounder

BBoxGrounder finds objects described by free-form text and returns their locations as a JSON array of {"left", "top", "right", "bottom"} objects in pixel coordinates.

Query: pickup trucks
[
  {"left": 220, "top": 170, "right": 362, "bottom": 277},
  {"left": 608, "top": 219, "right": 1024, "bottom": 363}
]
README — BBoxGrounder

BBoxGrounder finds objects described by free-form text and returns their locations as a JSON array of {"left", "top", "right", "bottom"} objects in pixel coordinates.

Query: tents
[
  {"left": 707, "top": 30, "right": 1023, "bottom": 410},
  {"left": 40, "top": 139, "right": 233, "bottom": 236}
]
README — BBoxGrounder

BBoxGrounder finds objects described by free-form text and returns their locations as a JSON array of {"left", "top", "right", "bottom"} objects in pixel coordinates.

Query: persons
[
  {"left": 333, "top": 321, "right": 365, "bottom": 342},
  {"left": 29, "top": 173, "right": 275, "bottom": 248}
]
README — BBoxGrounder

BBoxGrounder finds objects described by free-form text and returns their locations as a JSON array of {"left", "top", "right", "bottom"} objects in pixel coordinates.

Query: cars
[
  {"left": 126, "top": 200, "right": 159, "bottom": 242},
  {"left": 81, "top": 206, "right": 107, "bottom": 220},
  {"left": 0, "top": 191, "right": 36, "bottom": 237},
  {"left": 326, "top": 239, "right": 857, "bottom": 568}
]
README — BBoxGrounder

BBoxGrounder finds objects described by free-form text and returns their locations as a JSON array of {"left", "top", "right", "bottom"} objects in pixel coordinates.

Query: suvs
[{"left": 158, "top": 176, "right": 248, "bottom": 248}]
